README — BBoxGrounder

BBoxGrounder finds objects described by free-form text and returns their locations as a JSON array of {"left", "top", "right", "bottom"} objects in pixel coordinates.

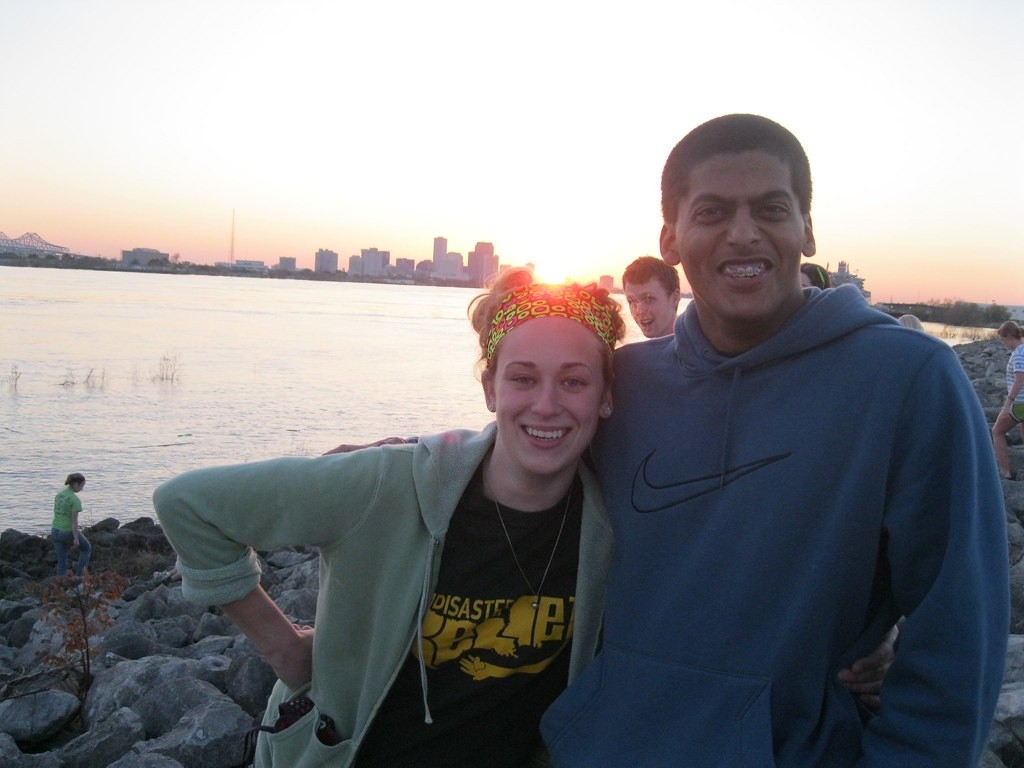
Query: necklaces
[{"left": 484, "top": 463, "right": 571, "bottom": 609}]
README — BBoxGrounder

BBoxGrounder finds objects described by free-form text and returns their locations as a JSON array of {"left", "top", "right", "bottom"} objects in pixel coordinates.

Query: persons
[
  {"left": 992, "top": 321, "right": 1024, "bottom": 480},
  {"left": 799, "top": 263, "right": 829, "bottom": 290},
  {"left": 51, "top": 473, "right": 91, "bottom": 591},
  {"left": 153, "top": 267, "right": 898, "bottom": 768},
  {"left": 324, "top": 113, "right": 1011, "bottom": 768},
  {"left": 622, "top": 257, "right": 681, "bottom": 338},
  {"left": 898, "top": 314, "right": 924, "bottom": 332}
]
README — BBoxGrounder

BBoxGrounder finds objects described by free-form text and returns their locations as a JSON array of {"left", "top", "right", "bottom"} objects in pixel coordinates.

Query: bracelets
[{"left": 1007, "top": 396, "right": 1014, "bottom": 401}]
